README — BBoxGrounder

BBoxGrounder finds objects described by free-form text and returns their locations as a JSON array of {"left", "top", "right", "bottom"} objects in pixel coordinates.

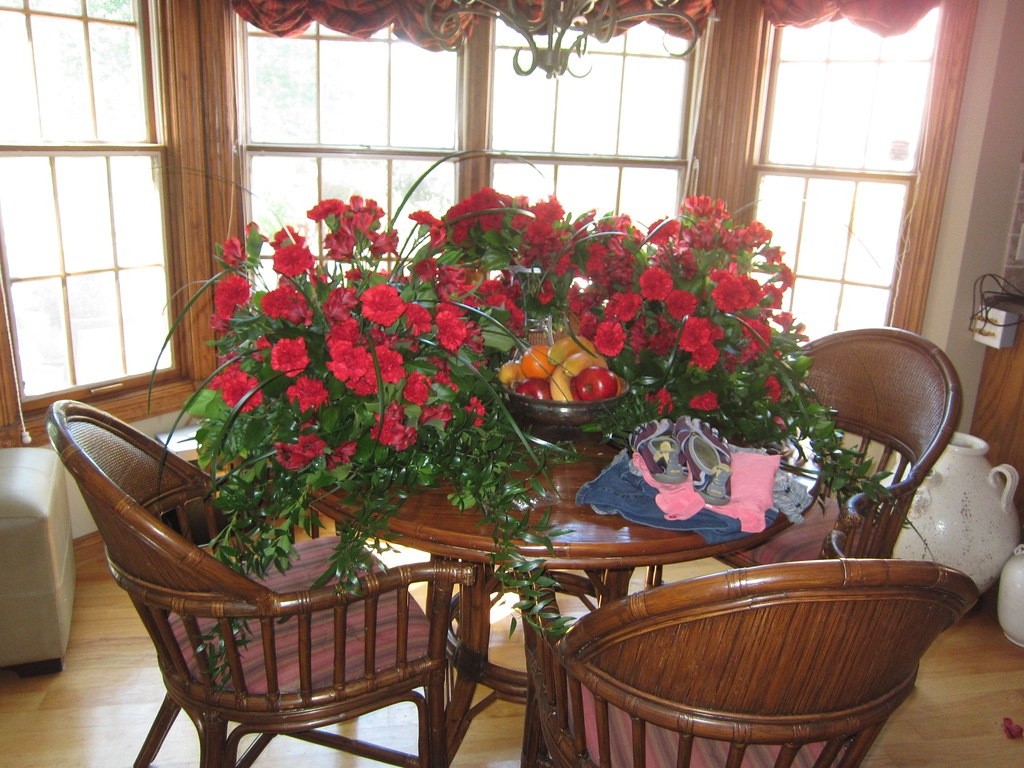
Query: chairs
[
  {"left": 646, "top": 326, "right": 962, "bottom": 592},
  {"left": 520, "top": 559, "right": 979, "bottom": 768},
  {"left": 45, "top": 401, "right": 478, "bottom": 768}
]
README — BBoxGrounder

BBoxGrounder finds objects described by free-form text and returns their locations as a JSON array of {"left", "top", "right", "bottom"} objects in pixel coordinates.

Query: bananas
[
  {"left": 499, "top": 363, "right": 526, "bottom": 389},
  {"left": 547, "top": 336, "right": 608, "bottom": 402}
]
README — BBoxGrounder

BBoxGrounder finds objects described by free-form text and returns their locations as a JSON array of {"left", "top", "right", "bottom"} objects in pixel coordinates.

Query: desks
[{"left": 294, "top": 422, "right": 823, "bottom": 768}]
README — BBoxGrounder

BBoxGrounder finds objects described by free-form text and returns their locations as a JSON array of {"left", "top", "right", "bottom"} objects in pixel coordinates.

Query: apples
[
  {"left": 517, "top": 377, "right": 551, "bottom": 401},
  {"left": 576, "top": 367, "right": 618, "bottom": 401}
]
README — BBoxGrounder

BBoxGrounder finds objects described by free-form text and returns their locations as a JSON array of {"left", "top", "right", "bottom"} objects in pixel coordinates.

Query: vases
[{"left": 878, "top": 433, "right": 1019, "bottom": 595}]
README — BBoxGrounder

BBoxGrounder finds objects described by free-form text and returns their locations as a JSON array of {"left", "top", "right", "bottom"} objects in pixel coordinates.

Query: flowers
[{"left": 147, "top": 160, "right": 939, "bottom": 657}]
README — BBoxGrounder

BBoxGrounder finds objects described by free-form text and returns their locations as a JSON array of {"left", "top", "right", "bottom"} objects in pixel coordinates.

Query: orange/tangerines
[{"left": 521, "top": 344, "right": 555, "bottom": 378}]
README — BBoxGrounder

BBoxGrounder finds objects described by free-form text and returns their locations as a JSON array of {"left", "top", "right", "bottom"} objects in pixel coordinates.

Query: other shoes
[
  {"left": 675, "top": 416, "right": 732, "bottom": 505},
  {"left": 629, "top": 419, "right": 688, "bottom": 483}
]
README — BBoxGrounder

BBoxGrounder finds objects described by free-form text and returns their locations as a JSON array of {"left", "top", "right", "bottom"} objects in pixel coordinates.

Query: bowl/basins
[{"left": 505, "top": 375, "right": 631, "bottom": 446}]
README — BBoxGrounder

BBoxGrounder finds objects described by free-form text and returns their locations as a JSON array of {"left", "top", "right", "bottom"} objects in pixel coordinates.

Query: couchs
[{"left": 0, "top": 446, "right": 75, "bottom": 677}]
